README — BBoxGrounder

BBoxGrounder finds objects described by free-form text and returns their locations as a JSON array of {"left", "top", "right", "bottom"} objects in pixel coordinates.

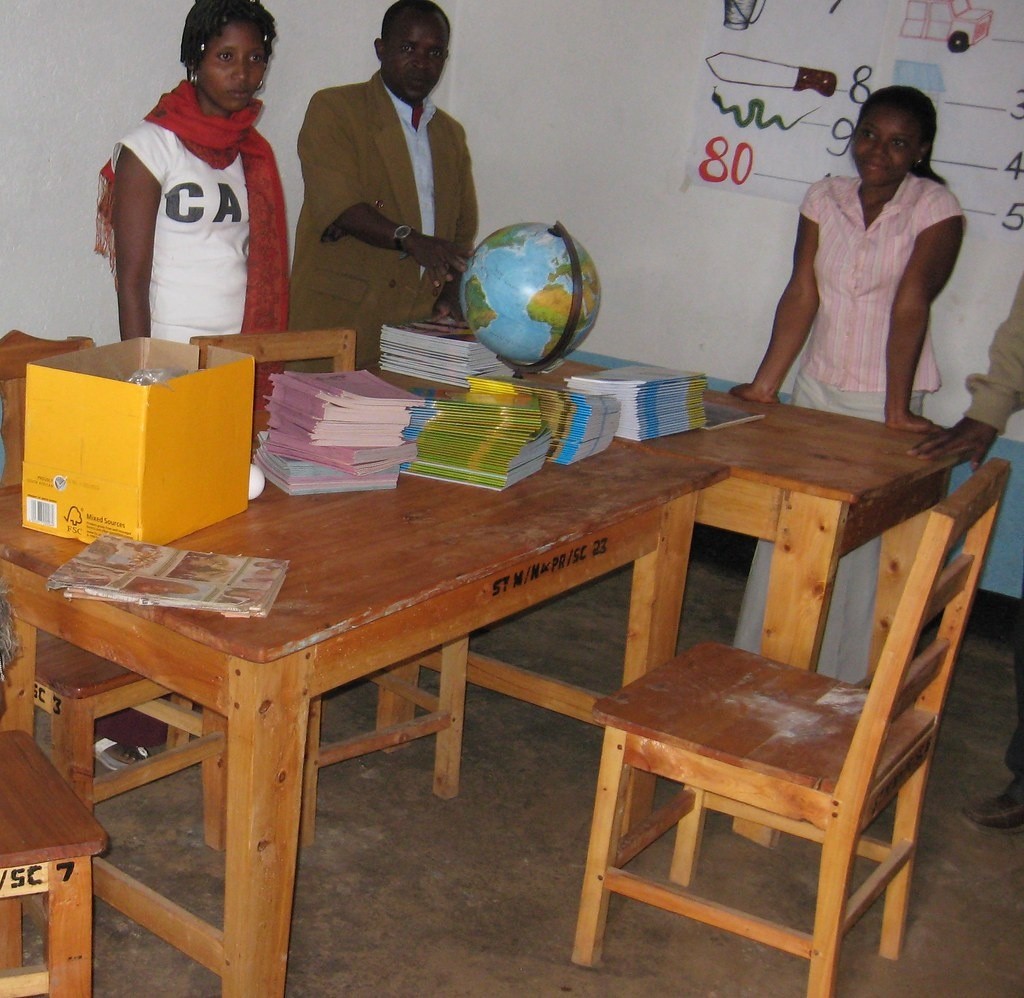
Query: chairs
[
  {"left": 0, "top": 728, "right": 113, "bottom": 998},
  {"left": 569, "top": 456, "right": 1012, "bottom": 997},
  {"left": 0, "top": 326, "right": 233, "bottom": 853},
  {"left": 191, "top": 329, "right": 470, "bottom": 852}
]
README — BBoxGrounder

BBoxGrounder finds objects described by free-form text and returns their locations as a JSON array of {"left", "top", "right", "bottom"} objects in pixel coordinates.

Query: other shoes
[
  {"left": 92, "top": 733, "right": 148, "bottom": 772},
  {"left": 962, "top": 793, "right": 1024, "bottom": 836}
]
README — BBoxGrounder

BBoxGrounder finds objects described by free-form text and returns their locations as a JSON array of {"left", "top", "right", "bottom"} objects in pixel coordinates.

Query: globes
[{"left": 459, "top": 220, "right": 601, "bottom": 379}]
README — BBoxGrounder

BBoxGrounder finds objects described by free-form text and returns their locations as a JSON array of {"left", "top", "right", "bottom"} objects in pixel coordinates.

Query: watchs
[{"left": 394, "top": 224, "right": 415, "bottom": 251}]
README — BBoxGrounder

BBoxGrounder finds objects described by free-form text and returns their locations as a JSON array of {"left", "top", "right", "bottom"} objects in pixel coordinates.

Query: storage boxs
[{"left": 21, "top": 337, "right": 256, "bottom": 548}]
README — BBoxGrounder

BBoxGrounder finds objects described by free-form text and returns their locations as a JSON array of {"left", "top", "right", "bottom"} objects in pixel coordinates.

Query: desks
[
  {"left": 0, "top": 436, "right": 730, "bottom": 998},
  {"left": 373, "top": 357, "right": 972, "bottom": 852}
]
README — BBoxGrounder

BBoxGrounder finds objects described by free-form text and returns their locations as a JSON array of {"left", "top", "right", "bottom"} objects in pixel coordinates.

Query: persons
[
  {"left": 55, "top": 537, "right": 283, "bottom": 607},
  {"left": 94, "top": 0, "right": 289, "bottom": 771},
  {"left": 286, "top": 1, "right": 479, "bottom": 373},
  {"left": 728, "top": 84, "right": 967, "bottom": 685},
  {"left": 909, "top": 272, "right": 1024, "bottom": 834}
]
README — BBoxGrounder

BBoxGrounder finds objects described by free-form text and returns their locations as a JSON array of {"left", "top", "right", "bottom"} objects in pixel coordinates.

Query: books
[
  {"left": 253, "top": 314, "right": 710, "bottom": 496},
  {"left": 45, "top": 536, "right": 290, "bottom": 619}
]
